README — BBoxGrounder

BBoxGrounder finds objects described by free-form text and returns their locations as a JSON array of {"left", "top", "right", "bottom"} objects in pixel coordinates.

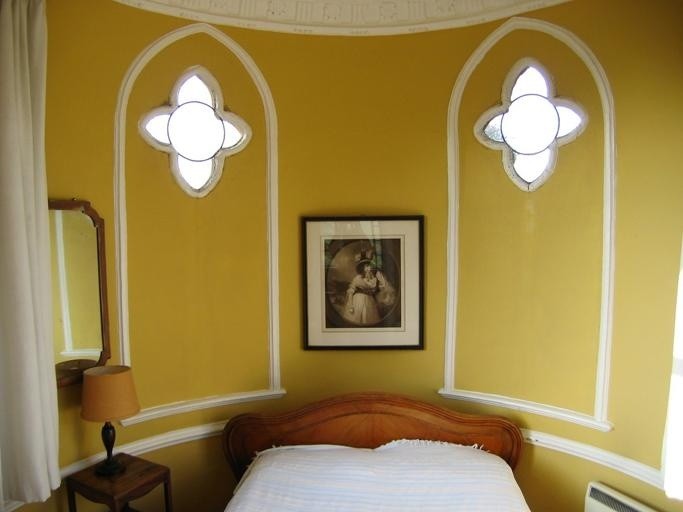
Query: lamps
[
  {"left": 54, "top": 359, "right": 97, "bottom": 381},
  {"left": 79, "top": 365, "right": 140, "bottom": 476}
]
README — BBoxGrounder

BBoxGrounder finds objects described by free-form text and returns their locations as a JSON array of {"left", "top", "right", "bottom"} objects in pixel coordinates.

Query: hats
[{"left": 356, "top": 258, "right": 377, "bottom": 276}]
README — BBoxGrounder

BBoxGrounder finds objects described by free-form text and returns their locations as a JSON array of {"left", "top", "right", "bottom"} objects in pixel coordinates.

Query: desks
[{"left": 63, "top": 452, "right": 172, "bottom": 510}]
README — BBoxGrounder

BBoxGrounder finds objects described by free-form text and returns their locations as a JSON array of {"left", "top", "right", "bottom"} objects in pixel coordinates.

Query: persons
[{"left": 341, "top": 252, "right": 388, "bottom": 326}]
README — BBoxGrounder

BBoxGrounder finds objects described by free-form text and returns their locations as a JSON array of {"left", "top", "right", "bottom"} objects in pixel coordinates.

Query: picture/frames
[{"left": 299, "top": 215, "right": 424, "bottom": 350}]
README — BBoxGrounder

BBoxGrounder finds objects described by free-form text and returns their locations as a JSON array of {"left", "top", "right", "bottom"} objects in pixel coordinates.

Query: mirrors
[{"left": 47, "top": 198, "right": 111, "bottom": 388}]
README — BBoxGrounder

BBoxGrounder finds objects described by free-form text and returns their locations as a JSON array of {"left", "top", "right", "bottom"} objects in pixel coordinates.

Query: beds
[{"left": 221, "top": 391, "right": 533, "bottom": 512}]
